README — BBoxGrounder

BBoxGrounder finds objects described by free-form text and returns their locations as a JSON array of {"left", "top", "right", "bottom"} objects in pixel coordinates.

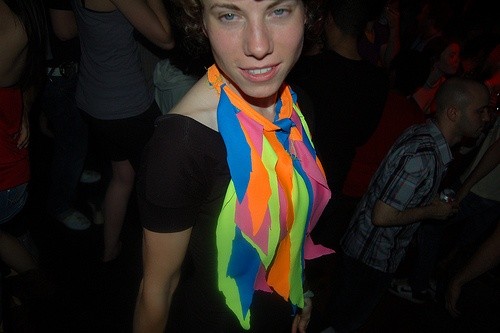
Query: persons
[
  {"left": 385, "top": 117, "right": 500, "bottom": 319},
  {"left": 0, "top": 0, "right": 176, "bottom": 309},
  {"left": 291, "top": 0, "right": 500, "bottom": 244},
  {"left": 444, "top": 223, "right": 500, "bottom": 316},
  {"left": 322, "top": 78, "right": 490, "bottom": 333},
  {"left": 132, "top": 0, "right": 337, "bottom": 333}
]
